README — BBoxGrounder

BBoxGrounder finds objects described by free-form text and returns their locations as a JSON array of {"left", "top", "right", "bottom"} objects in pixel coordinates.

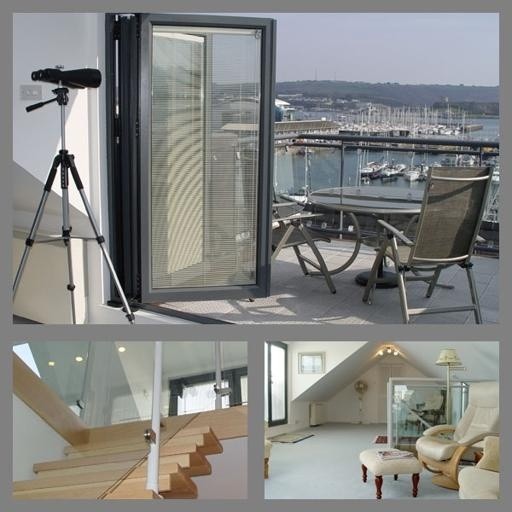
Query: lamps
[
  {"left": 394, "top": 351, "right": 398, "bottom": 356},
  {"left": 379, "top": 350, "right": 383, "bottom": 356},
  {"left": 387, "top": 347, "right": 391, "bottom": 354},
  {"left": 435, "top": 349, "right": 464, "bottom": 439}
]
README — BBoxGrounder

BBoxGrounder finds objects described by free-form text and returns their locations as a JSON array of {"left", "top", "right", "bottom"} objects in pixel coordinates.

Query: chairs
[
  {"left": 416, "top": 381, "right": 500, "bottom": 490},
  {"left": 406, "top": 394, "right": 445, "bottom": 435},
  {"left": 362, "top": 166, "right": 493, "bottom": 324},
  {"left": 241, "top": 149, "right": 336, "bottom": 302}
]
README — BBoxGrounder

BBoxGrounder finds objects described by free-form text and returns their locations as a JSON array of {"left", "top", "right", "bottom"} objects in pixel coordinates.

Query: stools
[{"left": 359, "top": 447, "right": 423, "bottom": 499}]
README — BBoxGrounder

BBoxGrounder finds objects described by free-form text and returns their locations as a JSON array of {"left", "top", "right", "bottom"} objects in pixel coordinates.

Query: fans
[{"left": 351, "top": 380, "right": 372, "bottom": 425}]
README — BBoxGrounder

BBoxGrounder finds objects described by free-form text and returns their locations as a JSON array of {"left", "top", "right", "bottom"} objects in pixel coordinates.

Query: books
[{"left": 377, "top": 451, "right": 413, "bottom": 459}]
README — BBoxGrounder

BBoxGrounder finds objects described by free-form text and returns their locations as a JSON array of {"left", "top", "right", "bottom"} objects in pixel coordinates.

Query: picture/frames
[{"left": 297, "top": 351, "right": 325, "bottom": 374}]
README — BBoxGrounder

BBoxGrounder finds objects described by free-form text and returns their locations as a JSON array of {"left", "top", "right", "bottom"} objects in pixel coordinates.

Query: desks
[{"left": 307, "top": 185, "right": 454, "bottom": 290}]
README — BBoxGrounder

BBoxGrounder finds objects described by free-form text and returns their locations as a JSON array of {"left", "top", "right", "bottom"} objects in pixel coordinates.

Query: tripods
[{"left": 12, "top": 82, "right": 136, "bottom": 324}]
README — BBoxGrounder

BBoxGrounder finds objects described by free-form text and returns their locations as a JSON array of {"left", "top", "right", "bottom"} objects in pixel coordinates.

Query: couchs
[{"left": 457, "top": 436, "right": 500, "bottom": 499}]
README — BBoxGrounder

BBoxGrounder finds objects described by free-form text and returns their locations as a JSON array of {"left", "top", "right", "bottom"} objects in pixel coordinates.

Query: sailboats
[{"left": 324, "top": 100, "right": 500, "bottom": 182}]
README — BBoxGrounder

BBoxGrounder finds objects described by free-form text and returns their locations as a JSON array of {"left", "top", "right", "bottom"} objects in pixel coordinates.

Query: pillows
[{"left": 475, "top": 436, "right": 500, "bottom": 472}]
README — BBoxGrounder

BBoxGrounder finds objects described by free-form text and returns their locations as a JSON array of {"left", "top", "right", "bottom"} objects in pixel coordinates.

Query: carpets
[
  {"left": 374, "top": 435, "right": 418, "bottom": 444},
  {"left": 269, "top": 432, "right": 315, "bottom": 443}
]
